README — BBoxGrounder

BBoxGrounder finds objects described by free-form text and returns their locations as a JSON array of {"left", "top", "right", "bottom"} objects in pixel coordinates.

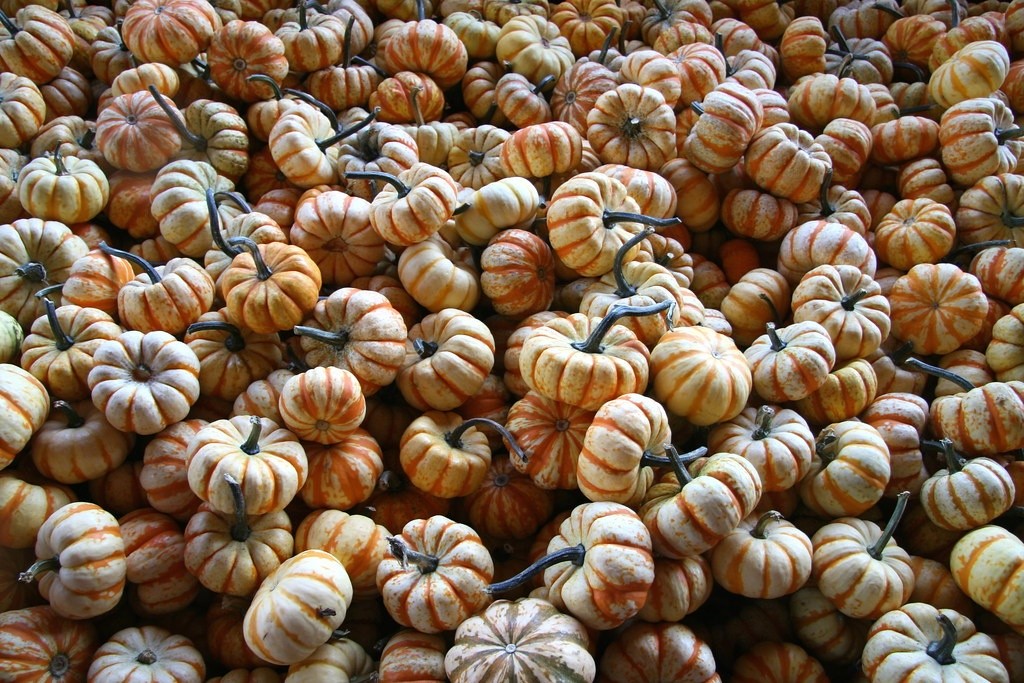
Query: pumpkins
[{"left": 0, "top": 0, "right": 1024, "bottom": 683}]
